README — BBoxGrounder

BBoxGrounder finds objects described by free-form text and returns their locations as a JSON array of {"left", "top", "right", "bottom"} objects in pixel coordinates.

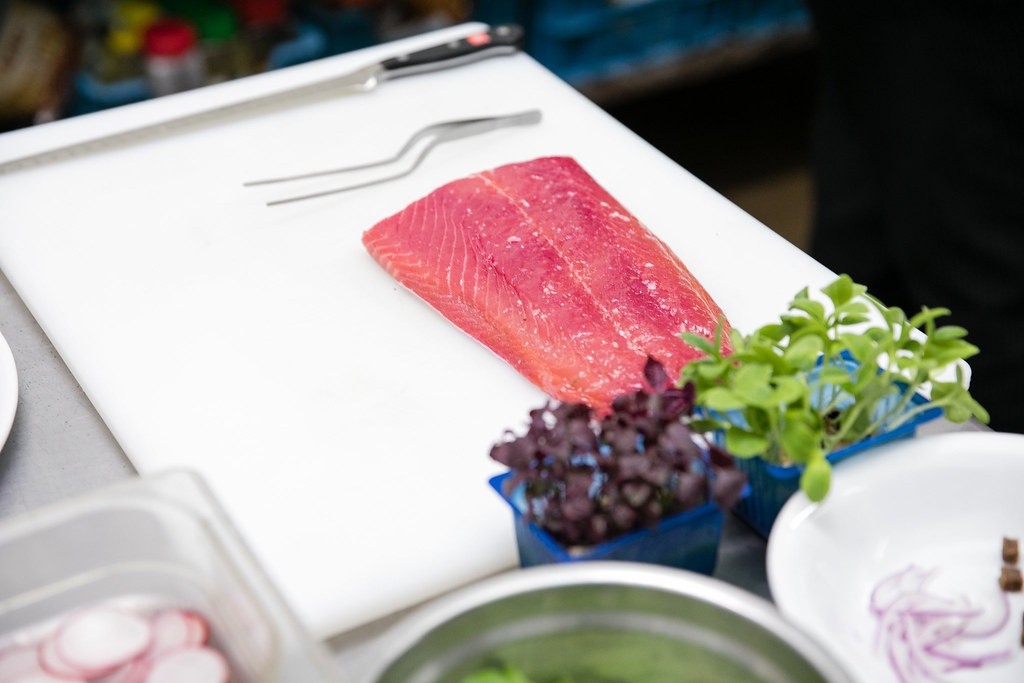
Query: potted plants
[
  {"left": 486, "top": 351, "right": 749, "bottom": 581},
  {"left": 674, "top": 271, "right": 990, "bottom": 540}
]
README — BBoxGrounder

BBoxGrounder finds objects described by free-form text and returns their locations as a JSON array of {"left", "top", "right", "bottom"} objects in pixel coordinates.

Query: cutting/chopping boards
[{"left": 0, "top": 20, "right": 971, "bottom": 645}]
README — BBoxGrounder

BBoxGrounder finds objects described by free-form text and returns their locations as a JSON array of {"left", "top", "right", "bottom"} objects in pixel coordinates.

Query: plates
[
  {"left": 0, "top": 328, "right": 18, "bottom": 448},
  {"left": 766, "top": 429, "right": 1024, "bottom": 683}
]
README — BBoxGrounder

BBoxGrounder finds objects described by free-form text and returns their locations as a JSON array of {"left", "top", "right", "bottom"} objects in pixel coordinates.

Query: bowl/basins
[{"left": 349, "top": 560, "right": 857, "bottom": 683}]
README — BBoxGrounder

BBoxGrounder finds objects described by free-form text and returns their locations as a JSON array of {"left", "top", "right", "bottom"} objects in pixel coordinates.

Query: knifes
[{"left": 0, "top": 25, "right": 524, "bottom": 178}]
